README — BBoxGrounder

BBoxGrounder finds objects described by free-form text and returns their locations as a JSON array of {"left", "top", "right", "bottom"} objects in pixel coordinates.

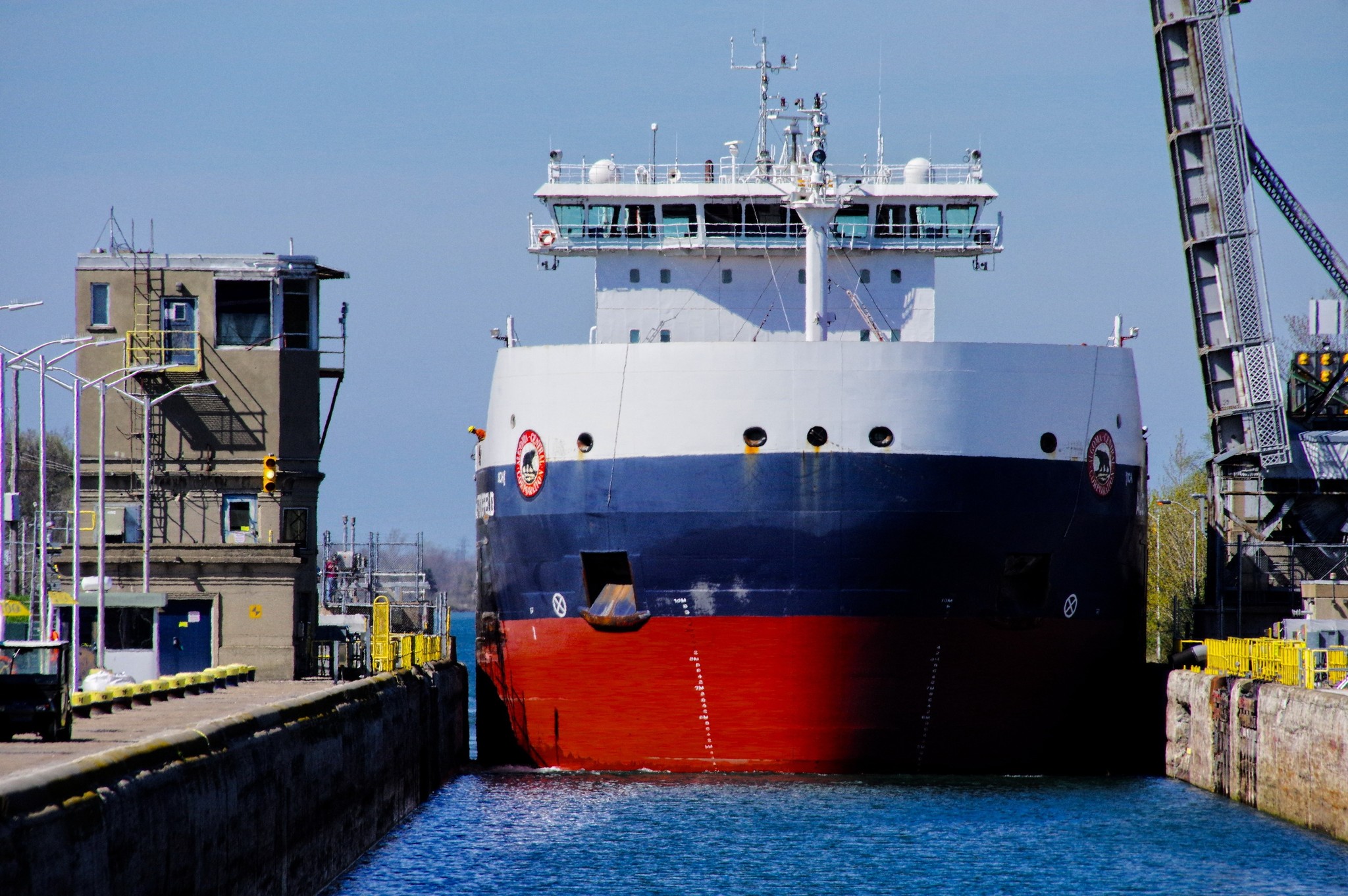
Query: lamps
[
  {"left": 489, "top": 328, "right": 508, "bottom": 346},
  {"left": 809, "top": 149, "right": 826, "bottom": 164},
  {"left": 1120, "top": 327, "right": 1139, "bottom": 347},
  {"left": 549, "top": 149, "right": 564, "bottom": 163},
  {"left": 967, "top": 149, "right": 982, "bottom": 165}
]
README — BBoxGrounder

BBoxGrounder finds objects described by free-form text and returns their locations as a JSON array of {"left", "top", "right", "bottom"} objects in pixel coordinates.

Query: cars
[{"left": 0, "top": 639, "right": 73, "bottom": 742}]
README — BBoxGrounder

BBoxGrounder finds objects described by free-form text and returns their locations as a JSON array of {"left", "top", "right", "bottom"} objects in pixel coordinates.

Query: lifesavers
[
  {"left": 538, "top": 230, "right": 556, "bottom": 246},
  {"left": 50, "top": 631, "right": 59, "bottom": 661},
  {"left": 0, "top": 654, "right": 17, "bottom": 675}
]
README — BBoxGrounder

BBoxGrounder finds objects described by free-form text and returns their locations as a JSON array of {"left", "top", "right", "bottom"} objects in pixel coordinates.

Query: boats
[
  {"left": 316, "top": 515, "right": 438, "bottom": 634},
  {"left": 468, "top": 32, "right": 1152, "bottom": 780}
]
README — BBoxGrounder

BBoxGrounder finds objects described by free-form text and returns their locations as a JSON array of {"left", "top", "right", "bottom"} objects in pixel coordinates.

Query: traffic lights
[{"left": 263, "top": 456, "right": 278, "bottom": 493}]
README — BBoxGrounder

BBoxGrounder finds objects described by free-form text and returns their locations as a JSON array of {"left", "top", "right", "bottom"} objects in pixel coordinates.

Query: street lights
[
  {"left": 1156, "top": 499, "right": 1197, "bottom": 601},
  {"left": 1, "top": 335, "right": 216, "bottom": 691}
]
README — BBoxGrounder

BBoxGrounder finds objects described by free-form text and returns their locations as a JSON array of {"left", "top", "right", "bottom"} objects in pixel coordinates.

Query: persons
[{"left": 325, "top": 559, "right": 337, "bottom": 602}]
